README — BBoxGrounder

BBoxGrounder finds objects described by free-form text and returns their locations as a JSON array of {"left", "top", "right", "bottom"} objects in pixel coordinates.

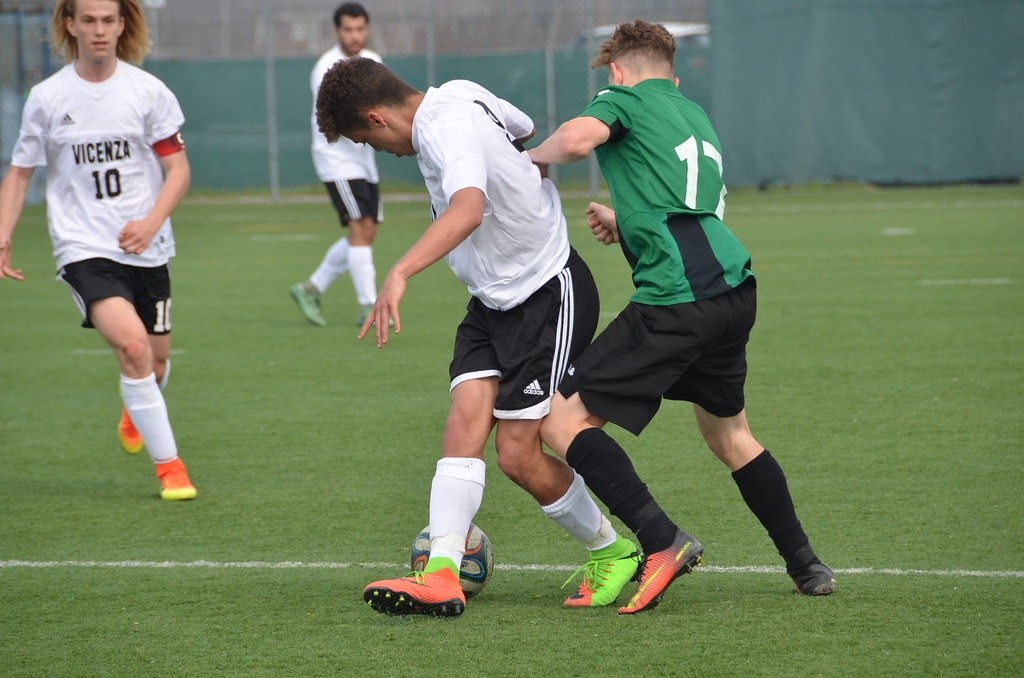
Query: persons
[
  {"left": 0, "top": 1, "right": 202, "bottom": 504},
  {"left": 314, "top": 58, "right": 647, "bottom": 619},
  {"left": 290, "top": 3, "right": 398, "bottom": 331},
  {"left": 519, "top": 18, "right": 840, "bottom": 614}
]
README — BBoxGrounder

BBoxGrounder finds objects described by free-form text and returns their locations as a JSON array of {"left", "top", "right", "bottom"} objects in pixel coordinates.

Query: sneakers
[
  {"left": 790, "top": 562, "right": 837, "bottom": 597},
  {"left": 363, "top": 572, "right": 467, "bottom": 619},
  {"left": 153, "top": 456, "right": 198, "bottom": 501},
  {"left": 563, "top": 539, "right": 644, "bottom": 608},
  {"left": 617, "top": 528, "right": 705, "bottom": 615},
  {"left": 357, "top": 304, "right": 395, "bottom": 328},
  {"left": 289, "top": 280, "right": 327, "bottom": 326},
  {"left": 117, "top": 379, "right": 144, "bottom": 456}
]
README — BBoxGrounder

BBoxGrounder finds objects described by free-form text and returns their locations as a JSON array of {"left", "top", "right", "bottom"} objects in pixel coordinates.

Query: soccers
[{"left": 410, "top": 521, "right": 494, "bottom": 601}]
